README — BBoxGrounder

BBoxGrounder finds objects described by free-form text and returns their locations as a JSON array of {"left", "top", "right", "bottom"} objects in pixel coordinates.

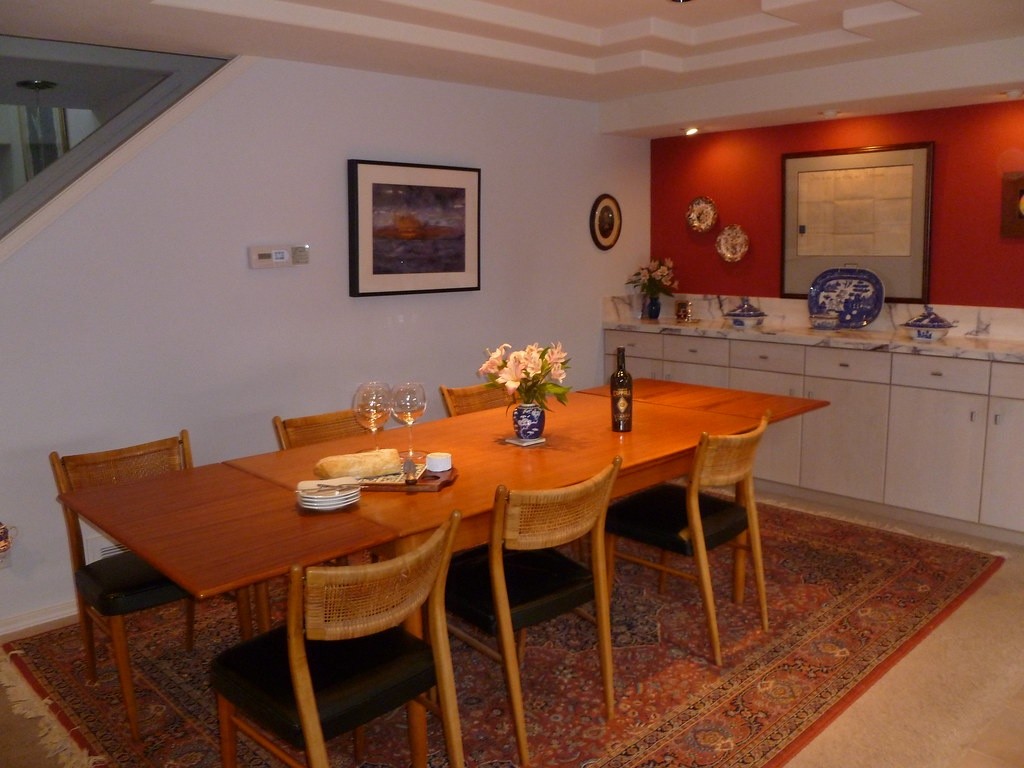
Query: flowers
[
  {"left": 478, "top": 341, "right": 574, "bottom": 417},
  {"left": 630, "top": 257, "right": 679, "bottom": 298}
]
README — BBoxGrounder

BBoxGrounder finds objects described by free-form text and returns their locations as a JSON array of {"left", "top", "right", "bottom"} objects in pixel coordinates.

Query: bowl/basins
[
  {"left": 724, "top": 300, "right": 767, "bottom": 329},
  {"left": 808, "top": 315, "right": 840, "bottom": 328},
  {"left": 899, "top": 308, "right": 958, "bottom": 341}
]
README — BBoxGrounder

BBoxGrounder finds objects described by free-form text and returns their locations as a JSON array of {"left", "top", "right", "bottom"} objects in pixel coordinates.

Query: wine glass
[
  {"left": 390, "top": 382, "right": 431, "bottom": 460},
  {"left": 352, "top": 382, "right": 392, "bottom": 453}
]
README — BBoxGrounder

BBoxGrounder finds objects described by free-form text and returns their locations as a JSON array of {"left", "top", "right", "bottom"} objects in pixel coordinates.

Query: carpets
[{"left": 1, "top": 491, "right": 1005, "bottom": 768}]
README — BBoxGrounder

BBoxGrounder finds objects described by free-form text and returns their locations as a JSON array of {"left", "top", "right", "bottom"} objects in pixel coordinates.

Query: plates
[
  {"left": 685, "top": 195, "right": 717, "bottom": 232},
  {"left": 807, "top": 268, "right": 884, "bottom": 329},
  {"left": 297, "top": 476, "right": 361, "bottom": 511},
  {"left": 715, "top": 225, "right": 750, "bottom": 262}
]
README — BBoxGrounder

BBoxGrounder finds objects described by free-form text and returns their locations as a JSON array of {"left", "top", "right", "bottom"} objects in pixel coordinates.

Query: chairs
[
  {"left": 272, "top": 406, "right": 384, "bottom": 566},
  {"left": 48, "top": 429, "right": 252, "bottom": 739},
  {"left": 208, "top": 510, "right": 461, "bottom": 768},
  {"left": 439, "top": 381, "right": 522, "bottom": 417},
  {"left": 428, "top": 456, "right": 623, "bottom": 768},
  {"left": 604, "top": 407, "right": 768, "bottom": 665}
]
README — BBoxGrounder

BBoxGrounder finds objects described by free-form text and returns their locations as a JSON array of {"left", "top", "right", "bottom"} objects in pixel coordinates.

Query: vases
[
  {"left": 513, "top": 403, "right": 545, "bottom": 439},
  {"left": 648, "top": 296, "right": 661, "bottom": 319}
]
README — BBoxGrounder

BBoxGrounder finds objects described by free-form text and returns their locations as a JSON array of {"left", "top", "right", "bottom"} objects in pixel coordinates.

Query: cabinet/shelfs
[{"left": 604, "top": 329, "right": 1024, "bottom": 533}]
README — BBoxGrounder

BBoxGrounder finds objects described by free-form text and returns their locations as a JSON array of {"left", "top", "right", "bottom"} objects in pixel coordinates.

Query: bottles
[{"left": 609, "top": 346, "right": 633, "bottom": 432}]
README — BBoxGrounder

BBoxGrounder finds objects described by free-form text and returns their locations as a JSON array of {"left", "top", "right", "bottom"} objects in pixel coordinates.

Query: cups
[{"left": 427, "top": 452, "right": 452, "bottom": 472}]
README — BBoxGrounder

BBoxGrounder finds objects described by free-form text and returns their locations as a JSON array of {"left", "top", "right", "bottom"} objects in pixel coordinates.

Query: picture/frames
[
  {"left": 780, "top": 141, "right": 933, "bottom": 305},
  {"left": 347, "top": 159, "right": 481, "bottom": 297},
  {"left": 1001, "top": 172, "right": 1024, "bottom": 236}
]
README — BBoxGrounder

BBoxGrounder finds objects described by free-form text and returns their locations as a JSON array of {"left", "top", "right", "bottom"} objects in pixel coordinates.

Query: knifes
[
  {"left": 295, "top": 483, "right": 369, "bottom": 494},
  {"left": 403, "top": 459, "right": 418, "bottom": 484}
]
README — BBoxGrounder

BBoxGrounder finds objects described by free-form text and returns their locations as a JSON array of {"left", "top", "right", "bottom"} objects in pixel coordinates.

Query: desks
[{"left": 57, "top": 379, "right": 829, "bottom": 768}]
